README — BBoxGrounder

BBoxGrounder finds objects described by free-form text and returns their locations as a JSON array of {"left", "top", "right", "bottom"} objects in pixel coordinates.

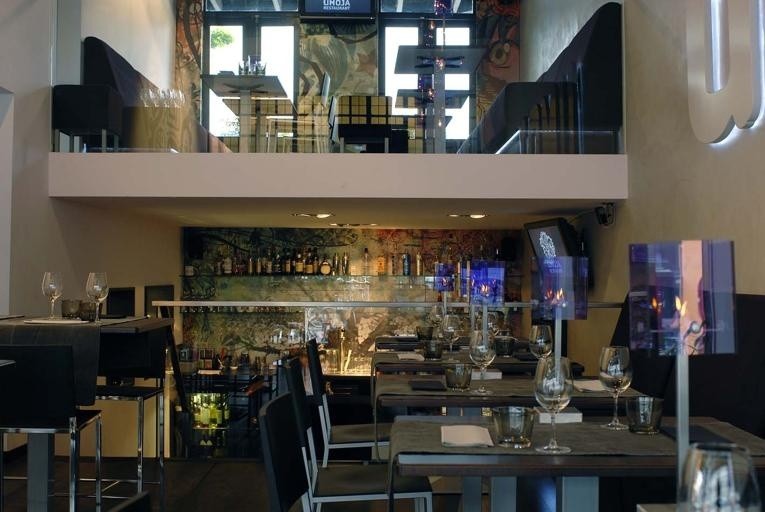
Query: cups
[
  {"left": 185, "top": 265, "right": 194, "bottom": 277},
  {"left": 445, "top": 364, "right": 472, "bottom": 392},
  {"left": 495, "top": 337, "right": 515, "bottom": 359},
  {"left": 491, "top": 407, "right": 536, "bottom": 449},
  {"left": 626, "top": 396, "right": 665, "bottom": 437},
  {"left": 422, "top": 340, "right": 443, "bottom": 362},
  {"left": 61, "top": 298, "right": 103, "bottom": 321},
  {"left": 238, "top": 61, "right": 266, "bottom": 76},
  {"left": 417, "top": 326, "right": 433, "bottom": 345}
]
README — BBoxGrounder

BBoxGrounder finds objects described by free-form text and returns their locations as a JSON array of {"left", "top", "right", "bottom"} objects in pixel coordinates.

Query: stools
[{"left": 52, "top": 84, "right": 122, "bottom": 153}]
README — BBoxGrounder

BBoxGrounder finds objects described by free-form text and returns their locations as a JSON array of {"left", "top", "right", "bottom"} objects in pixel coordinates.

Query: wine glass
[
  {"left": 85, "top": 271, "right": 109, "bottom": 324},
  {"left": 140, "top": 88, "right": 187, "bottom": 111},
  {"left": 529, "top": 325, "right": 553, "bottom": 360},
  {"left": 430, "top": 303, "right": 446, "bottom": 340},
  {"left": 534, "top": 356, "right": 575, "bottom": 453},
  {"left": 469, "top": 329, "right": 497, "bottom": 397},
  {"left": 442, "top": 322, "right": 461, "bottom": 363},
  {"left": 41, "top": 269, "right": 63, "bottom": 321},
  {"left": 598, "top": 345, "right": 635, "bottom": 432},
  {"left": 674, "top": 441, "right": 762, "bottom": 512}
]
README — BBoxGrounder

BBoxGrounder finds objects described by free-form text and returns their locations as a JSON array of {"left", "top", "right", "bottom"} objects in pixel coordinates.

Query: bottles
[
  {"left": 254, "top": 355, "right": 267, "bottom": 377},
  {"left": 212, "top": 247, "right": 354, "bottom": 276},
  {"left": 359, "top": 248, "right": 423, "bottom": 277},
  {"left": 192, "top": 394, "right": 229, "bottom": 428}
]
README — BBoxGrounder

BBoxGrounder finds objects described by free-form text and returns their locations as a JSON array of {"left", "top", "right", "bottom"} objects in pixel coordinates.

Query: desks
[
  {"left": 374, "top": 339, "right": 651, "bottom": 419},
  {"left": 384, "top": 412, "right": 762, "bottom": 510}
]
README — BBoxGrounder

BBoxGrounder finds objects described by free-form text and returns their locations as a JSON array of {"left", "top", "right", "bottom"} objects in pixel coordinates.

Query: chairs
[
  {"left": 266, "top": 72, "right": 339, "bottom": 152},
  {"left": 257, "top": 339, "right": 435, "bottom": 512},
  {"left": 2, "top": 329, "right": 166, "bottom": 511}
]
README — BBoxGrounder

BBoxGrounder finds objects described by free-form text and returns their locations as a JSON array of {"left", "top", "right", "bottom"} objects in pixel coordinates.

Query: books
[
  {"left": 533, "top": 406, "right": 583, "bottom": 424},
  {"left": 441, "top": 425, "right": 495, "bottom": 448},
  {"left": 565, "top": 380, "right": 608, "bottom": 393},
  {"left": 397, "top": 353, "right": 425, "bottom": 361},
  {"left": 471, "top": 368, "right": 502, "bottom": 380}
]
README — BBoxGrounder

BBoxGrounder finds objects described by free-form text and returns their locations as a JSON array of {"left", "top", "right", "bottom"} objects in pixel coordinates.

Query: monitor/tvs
[
  {"left": 524, "top": 217, "right": 595, "bottom": 292},
  {"left": 298, "top": 0, "right": 377, "bottom": 24}
]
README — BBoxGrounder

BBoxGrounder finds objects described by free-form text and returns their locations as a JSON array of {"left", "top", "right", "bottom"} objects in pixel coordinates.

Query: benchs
[
  {"left": 600, "top": 286, "right": 764, "bottom": 511},
  {"left": 86, "top": 36, "right": 231, "bottom": 152},
  {"left": 455, "top": 2, "right": 622, "bottom": 154}
]
